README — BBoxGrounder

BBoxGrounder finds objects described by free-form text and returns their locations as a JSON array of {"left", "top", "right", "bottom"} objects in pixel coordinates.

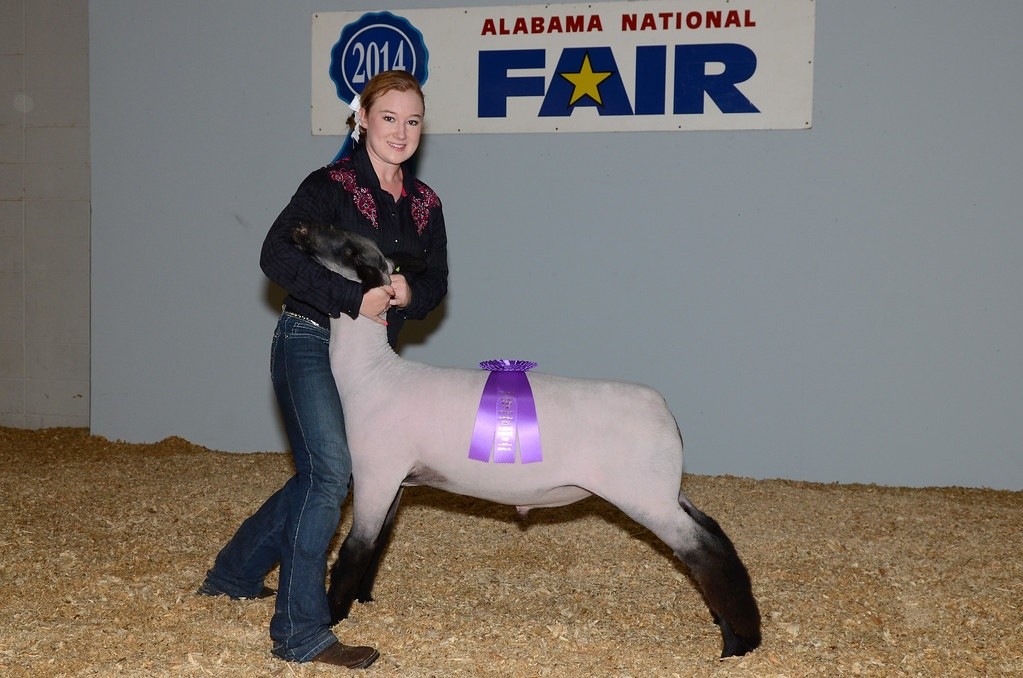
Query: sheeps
[{"left": 287, "top": 222, "right": 761, "bottom": 657}]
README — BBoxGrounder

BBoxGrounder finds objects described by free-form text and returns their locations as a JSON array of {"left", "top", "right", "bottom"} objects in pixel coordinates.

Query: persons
[{"left": 197, "top": 70, "right": 449, "bottom": 670}]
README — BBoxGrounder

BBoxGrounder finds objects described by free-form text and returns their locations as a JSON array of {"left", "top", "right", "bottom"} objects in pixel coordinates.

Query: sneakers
[
  {"left": 310, "top": 640, "right": 380, "bottom": 669},
  {"left": 197, "top": 578, "right": 274, "bottom": 600}
]
